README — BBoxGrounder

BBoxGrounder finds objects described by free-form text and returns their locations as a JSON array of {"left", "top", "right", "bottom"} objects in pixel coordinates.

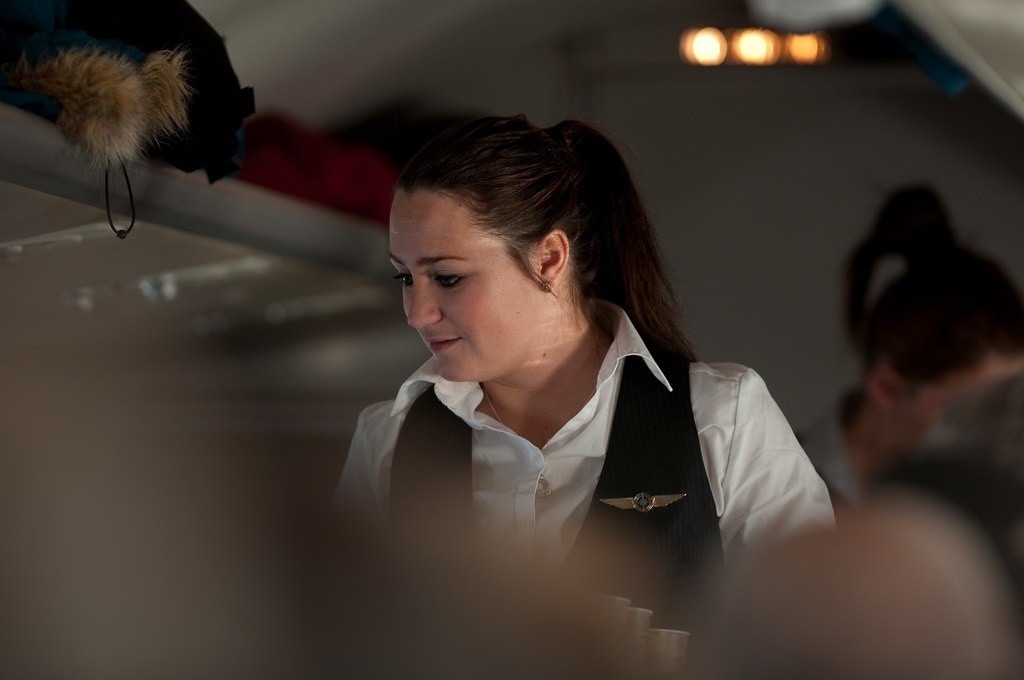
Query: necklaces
[{"left": 480, "top": 388, "right": 598, "bottom": 498}]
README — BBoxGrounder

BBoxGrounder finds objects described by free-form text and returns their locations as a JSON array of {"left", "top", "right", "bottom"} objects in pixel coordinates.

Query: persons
[
  {"left": 702, "top": 183, "right": 1024, "bottom": 679},
  {"left": 326, "top": 114, "right": 838, "bottom": 632}
]
